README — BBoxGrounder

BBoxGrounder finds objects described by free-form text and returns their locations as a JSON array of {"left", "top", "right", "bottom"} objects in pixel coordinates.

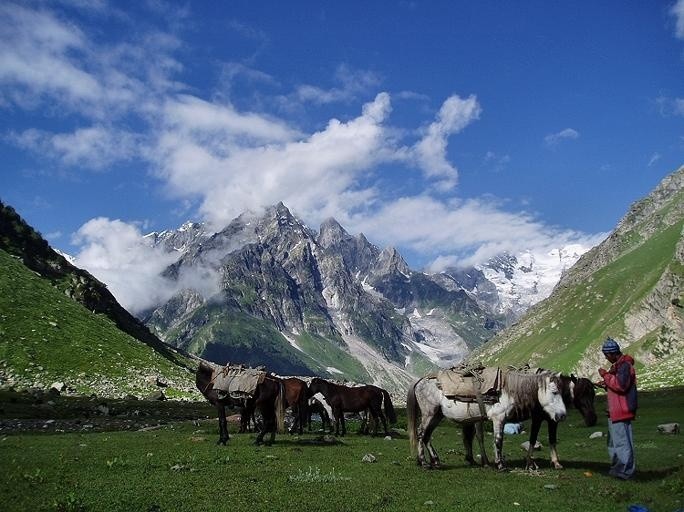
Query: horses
[
  {"left": 196, "top": 360, "right": 397, "bottom": 446},
  {"left": 406, "top": 366, "right": 567, "bottom": 472},
  {"left": 462, "top": 365, "right": 596, "bottom": 470}
]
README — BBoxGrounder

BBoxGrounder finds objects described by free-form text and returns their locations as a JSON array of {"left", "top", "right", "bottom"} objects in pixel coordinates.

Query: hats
[{"left": 602, "top": 337, "right": 619, "bottom": 353}]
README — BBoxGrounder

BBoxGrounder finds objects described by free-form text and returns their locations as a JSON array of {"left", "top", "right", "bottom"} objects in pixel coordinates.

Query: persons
[{"left": 597, "top": 338, "right": 637, "bottom": 479}]
